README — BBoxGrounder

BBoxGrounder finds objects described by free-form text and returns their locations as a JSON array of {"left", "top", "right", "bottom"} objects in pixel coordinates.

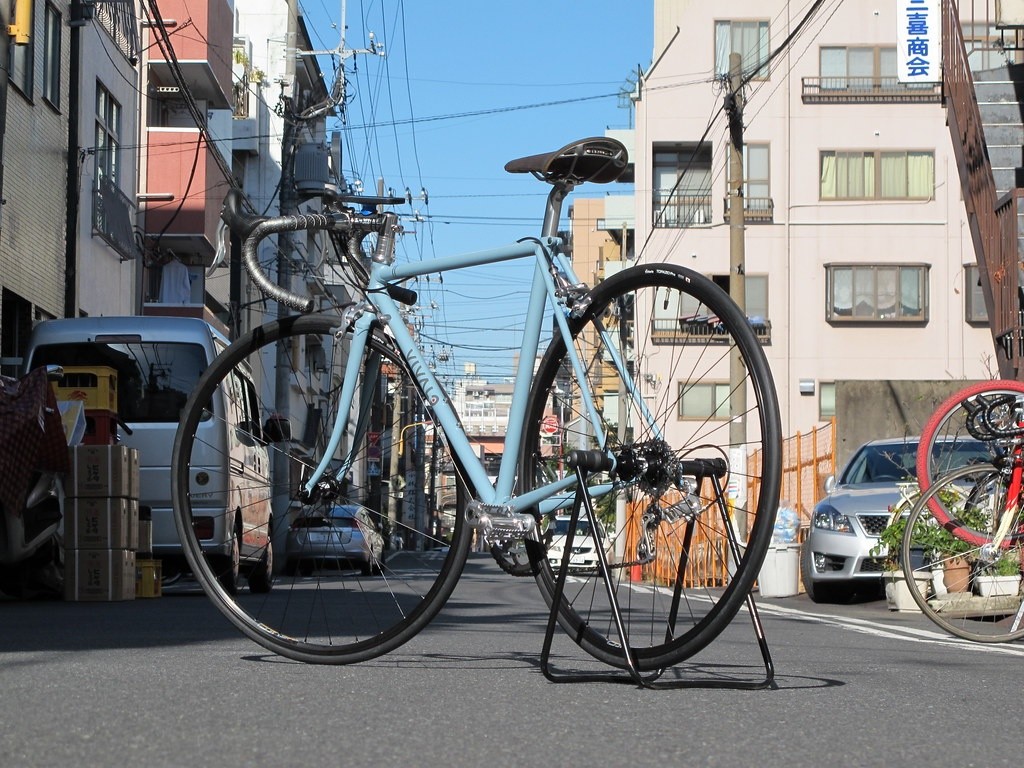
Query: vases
[
  {"left": 882, "top": 570, "right": 902, "bottom": 611},
  {"left": 894, "top": 571, "right": 936, "bottom": 614},
  {"left": 896, "top": 547, "right": 929, "bottom": 571}
]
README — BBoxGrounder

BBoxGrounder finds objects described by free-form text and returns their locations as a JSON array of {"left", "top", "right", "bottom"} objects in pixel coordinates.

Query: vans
[{"left": 25, "top": 315, "right": 291, "bottom": 598}]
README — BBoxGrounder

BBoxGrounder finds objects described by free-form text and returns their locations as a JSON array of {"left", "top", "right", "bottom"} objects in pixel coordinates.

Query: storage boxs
[{"left": 47, "top": 367, "right": 164, "bottom": 601}]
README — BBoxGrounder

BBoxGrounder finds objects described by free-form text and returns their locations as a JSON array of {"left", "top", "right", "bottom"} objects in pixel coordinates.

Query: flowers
[{"left": 868, "top": 483, "right": 963, "bottom": 568}]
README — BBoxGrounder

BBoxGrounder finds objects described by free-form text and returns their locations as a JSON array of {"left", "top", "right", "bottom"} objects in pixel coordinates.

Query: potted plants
[
  {"left": 939, "top": 540, "right": 972, "bottom": 592},
  {"left": 978, "top": 552, "right": 1022, "bottom": 597}
]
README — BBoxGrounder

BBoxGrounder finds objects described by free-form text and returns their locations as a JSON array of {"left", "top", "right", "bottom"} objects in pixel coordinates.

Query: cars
[
  {"left": 800, "top": 436, "right": 1004, "bottom": 604},
  {"left": 542, "top": 515, "right": 616, "bottom": 576},
  {"left": 286, "top": 503, "right": 386, "bottom": 577}
]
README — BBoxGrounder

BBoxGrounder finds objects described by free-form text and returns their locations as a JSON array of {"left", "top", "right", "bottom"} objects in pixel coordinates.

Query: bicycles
[
  {"left": 902, "top": 379, "right": 1024, "bottom": 644},
  {"left": 169, "top": 137, "right": 782, "bottom": 690}
]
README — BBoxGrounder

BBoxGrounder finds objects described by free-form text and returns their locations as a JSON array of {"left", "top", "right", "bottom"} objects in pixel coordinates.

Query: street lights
[{"left": 388, "top": 420, "right": 435, "bottom": 549}]
